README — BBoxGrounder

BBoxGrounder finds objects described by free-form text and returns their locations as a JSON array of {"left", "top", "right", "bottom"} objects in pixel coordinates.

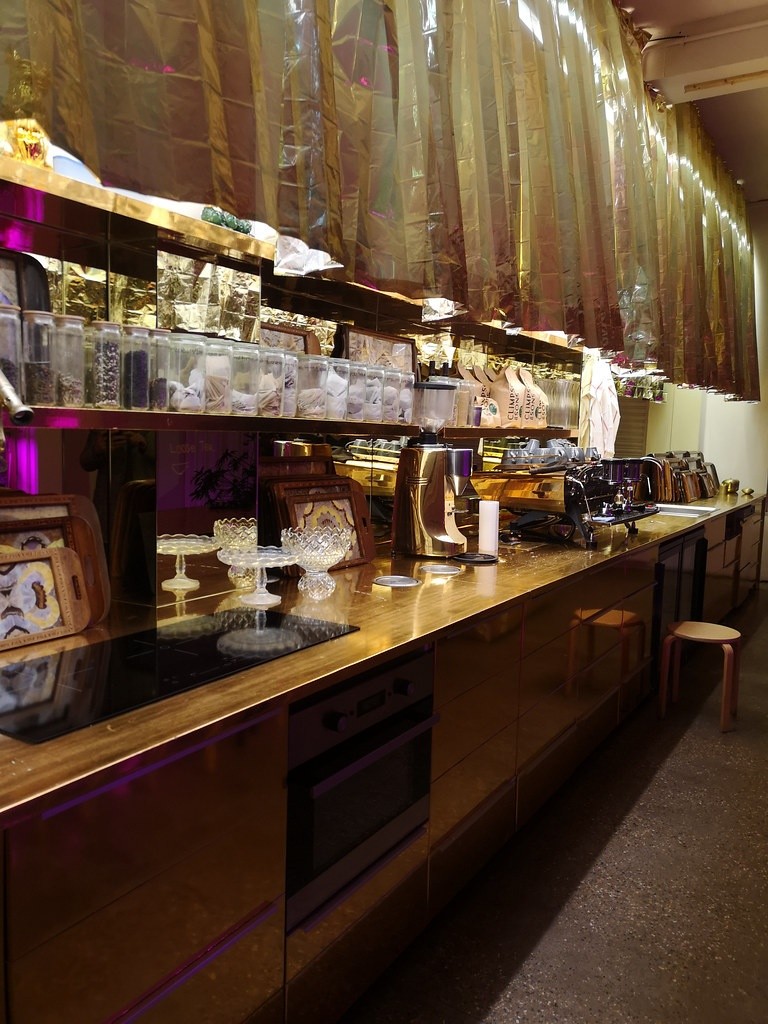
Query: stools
[
  {"left": 567, "top": 608, "right": 647, "bottom": 725},
  {"left": 657, "top": 623, "right": 743, "bottom": 734}
]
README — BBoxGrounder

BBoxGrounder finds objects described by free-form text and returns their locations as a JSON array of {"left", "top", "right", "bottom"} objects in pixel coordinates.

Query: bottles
[{"left": 0, "top": 304, "right": 482, "bottom": 427}]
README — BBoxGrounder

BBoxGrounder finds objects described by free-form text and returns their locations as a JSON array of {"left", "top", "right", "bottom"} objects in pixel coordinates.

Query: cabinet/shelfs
[
  {"left": 0, "top": 698, "right": 284, "bottom": 1024},
  {"left": 703, "top": 499, "right": 765, "bottom": 624}
]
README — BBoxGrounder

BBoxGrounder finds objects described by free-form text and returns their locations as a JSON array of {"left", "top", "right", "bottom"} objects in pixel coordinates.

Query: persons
[{"left": 79, "top": 429, "right": 158, "bottom": 561}]
